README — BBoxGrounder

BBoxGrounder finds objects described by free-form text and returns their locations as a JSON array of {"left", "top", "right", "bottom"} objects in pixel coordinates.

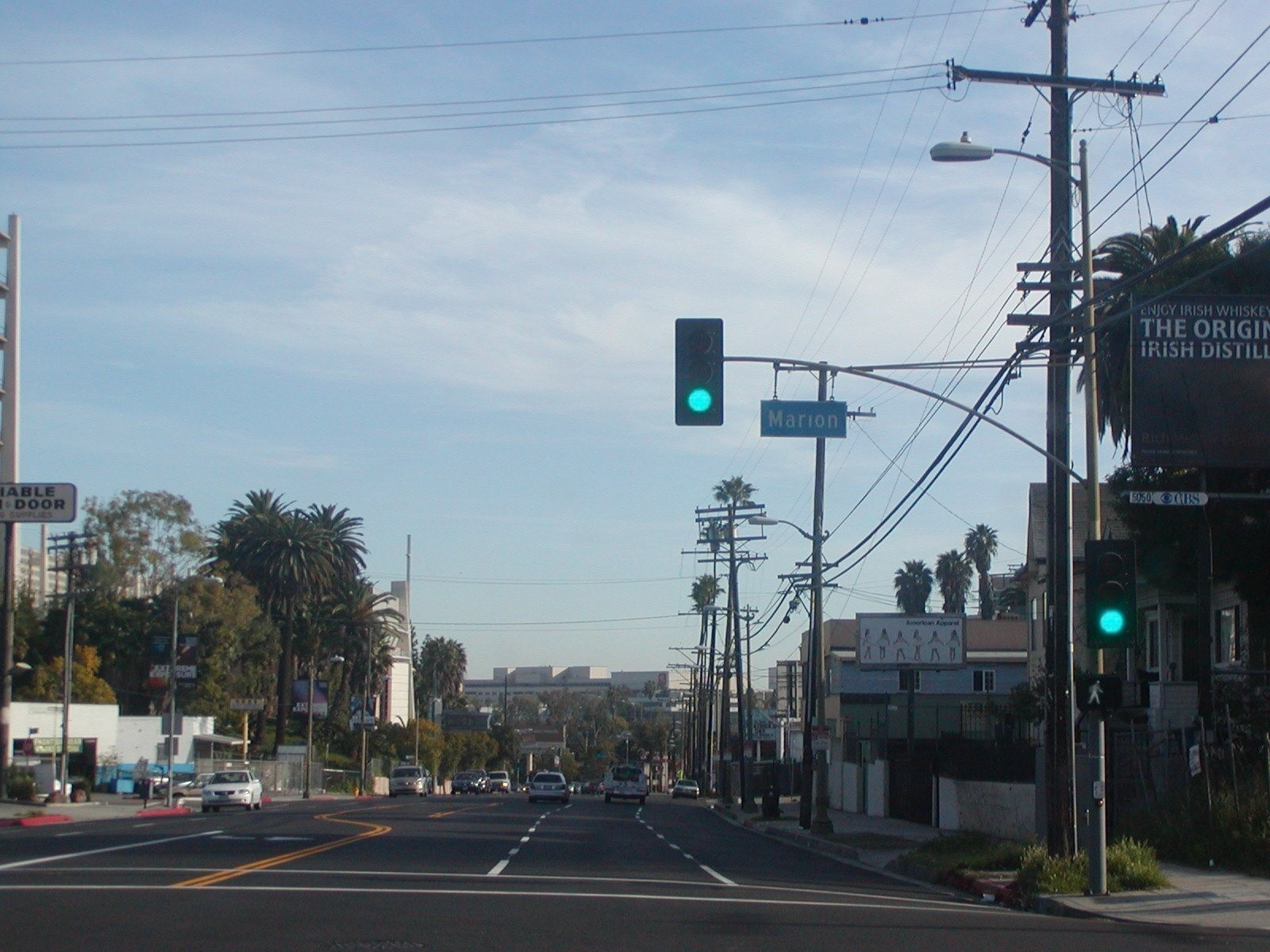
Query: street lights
[
  {"left": 683, "top": 643, "right": 735, "bottom": 802},
  {"left": 702, "top": 603, "right": 758, "bottom": 815},
  {"left": 615, "top": 733, "right": 629, "bottom": 764},
  {"left": 303, "top": 655, "right": 345, "bottom": 799},
  {"left": 748, "top": 514, "right": 836, "bottom": 836},
  {"left": 415, "top": 698, "right": 441, "bottom": 765},
  {"left": 165, "top": 576, "right": 224, "bottom": 810},
  {"left": 928, "top": 139, "right": 1110, "bottom": 897}
]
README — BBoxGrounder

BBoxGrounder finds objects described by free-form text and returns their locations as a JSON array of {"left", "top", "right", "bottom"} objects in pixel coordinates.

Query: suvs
[{"left": 487, "top": 770, "right": 511, "bottom": 794}]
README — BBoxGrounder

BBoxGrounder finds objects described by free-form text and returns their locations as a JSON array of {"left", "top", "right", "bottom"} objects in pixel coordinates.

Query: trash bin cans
[{"left": 140, "top": 779, "right": 154, "bottom": 800}]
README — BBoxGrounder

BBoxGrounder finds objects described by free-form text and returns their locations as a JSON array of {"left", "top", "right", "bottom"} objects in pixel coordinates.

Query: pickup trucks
[{"left": 602, "top": 763, "right": 650, "bottom": 805}]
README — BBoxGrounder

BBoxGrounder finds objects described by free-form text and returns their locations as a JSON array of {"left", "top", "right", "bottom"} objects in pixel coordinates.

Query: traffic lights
[
  {"left": 674, "top": 318, "right": 723, "bottom": 427},
  {"left": 1084, "top": 536, "right": 1140, "bottom": 651}
]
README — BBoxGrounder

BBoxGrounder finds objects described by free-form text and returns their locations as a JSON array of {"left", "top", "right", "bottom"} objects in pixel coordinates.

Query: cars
[
  {"left": 527, "top": 771, "right": 570, "bottom": 804},
  {"left": 567, "top": 780, "right": 605, "bottom": 795},
  {"left": 135, "top": 769, "right": 217, "bottom": 803},
  {"left": 671, "top": 779, "right": 700, "bottom": 800},
  {"left": 199, "top": 770, "right": 263, "bottom": 813},
  {"left": 450, "top": 769, "right": 493, "bottom": 795}
]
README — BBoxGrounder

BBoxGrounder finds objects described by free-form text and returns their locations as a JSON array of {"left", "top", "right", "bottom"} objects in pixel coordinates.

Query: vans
[{"left": 388, "top": 765, "right": 433, "bottom": 799}]
